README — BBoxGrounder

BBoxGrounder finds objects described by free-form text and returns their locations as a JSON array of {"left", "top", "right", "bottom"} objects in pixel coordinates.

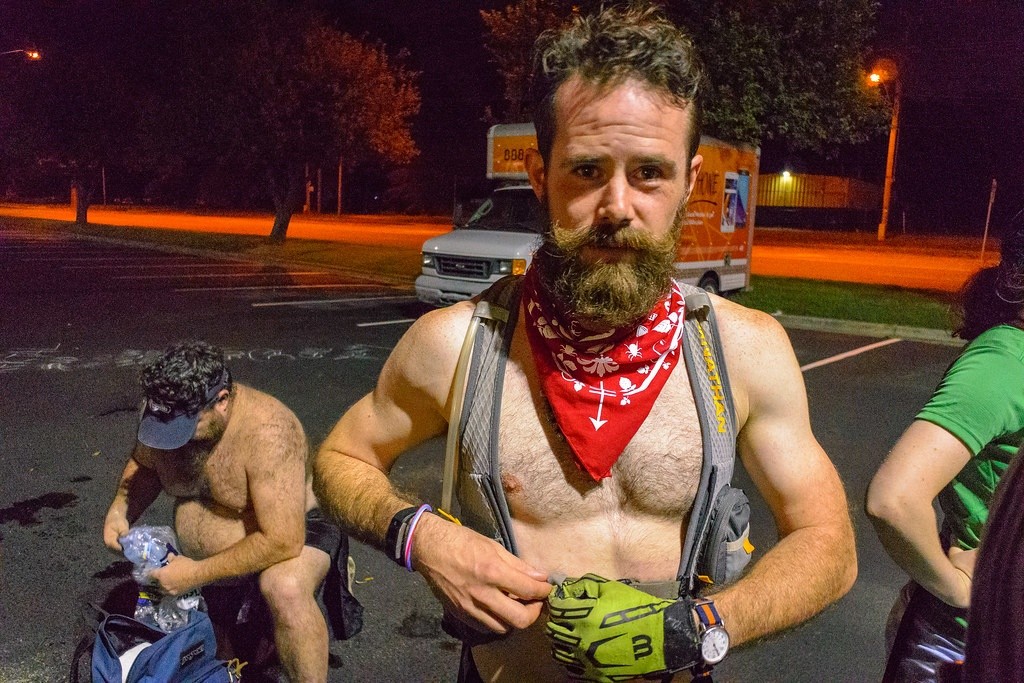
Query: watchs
[{"left": 693, "top": 594, "right": 731, "bottom": 679}]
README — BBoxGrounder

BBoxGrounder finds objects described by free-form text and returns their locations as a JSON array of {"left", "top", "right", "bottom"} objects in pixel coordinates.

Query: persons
[
  {"left": 312, "top": 1, "right": 856, "bottom": 683},
  {"left": 865, "top": 211, "right": 1024, "bottom": 682},
  {"left": 102, "top": 341, "right": 363, "bottom": 683}
]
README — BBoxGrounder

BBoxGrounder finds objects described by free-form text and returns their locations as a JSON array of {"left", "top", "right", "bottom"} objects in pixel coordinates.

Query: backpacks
[{"left": 71, "top": 602, "right": 240, "bottom": 683}]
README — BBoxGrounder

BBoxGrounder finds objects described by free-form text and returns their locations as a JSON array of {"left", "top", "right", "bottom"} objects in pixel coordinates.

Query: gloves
[{"left": 546, "top": 574, "right": 703, "bottom": 683}]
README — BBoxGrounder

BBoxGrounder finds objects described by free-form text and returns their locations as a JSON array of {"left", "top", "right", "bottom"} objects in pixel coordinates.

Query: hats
[{"left": 137, "top": 367, "right": 233, "bottom": 451}]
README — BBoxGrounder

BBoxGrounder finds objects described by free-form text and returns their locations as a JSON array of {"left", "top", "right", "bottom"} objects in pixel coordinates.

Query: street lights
[{"left": 871, "top": 72, "right": 897, "bottom": 242}]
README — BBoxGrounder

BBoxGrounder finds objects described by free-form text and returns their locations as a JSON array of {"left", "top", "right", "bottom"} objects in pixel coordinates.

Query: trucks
[{"left": 413, "top": 123, "right": 764, "bottom": 310}]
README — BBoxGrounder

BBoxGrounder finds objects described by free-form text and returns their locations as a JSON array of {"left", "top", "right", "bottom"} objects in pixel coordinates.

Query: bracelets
[{"left": 385, "top": 504, "right": 432, "bottom": 574}]
